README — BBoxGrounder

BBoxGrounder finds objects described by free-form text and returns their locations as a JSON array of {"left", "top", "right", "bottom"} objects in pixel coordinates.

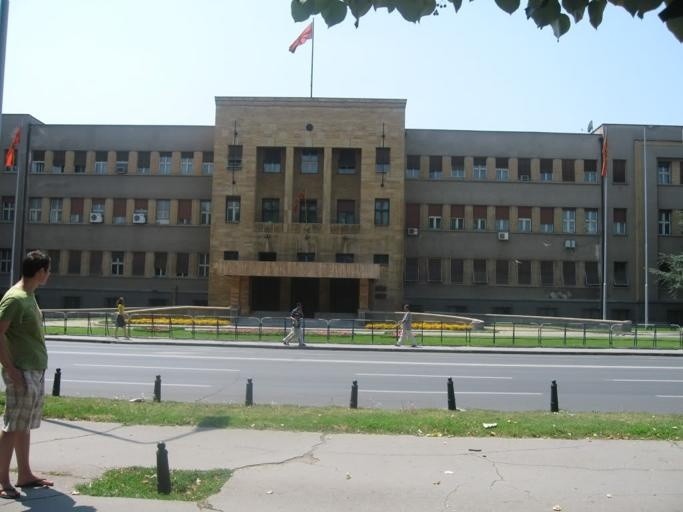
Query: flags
[
  {"left": 601, "top": 130, "right": 609, "bottom": 176},
  {"left": 289, "top": 23, "right": 311, "bottom": 56},
  {"left": 4, "top": 127, "right": 19, "bottom": 168}
]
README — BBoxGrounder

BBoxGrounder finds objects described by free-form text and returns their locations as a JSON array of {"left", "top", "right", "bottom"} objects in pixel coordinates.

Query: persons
[
  {"left": 0, "top": 244, "right": 55, "bottom": 500},
  {"left": 394, "top": 303, "right": 416, "bottom": 348},
  {"left": 111, "top": 296, "right": 130, "bottom": 339},
  {"left": 282, "top": 301, "right": 306, "bottom": 347}
]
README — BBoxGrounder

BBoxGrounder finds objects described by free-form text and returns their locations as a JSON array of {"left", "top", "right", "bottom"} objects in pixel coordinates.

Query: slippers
[
  {"left": 15, "top": 480, "right": 52, "bottom": 489},
  {"left": 0, "top": 489, "right": 20, "bottom": 500}
]
